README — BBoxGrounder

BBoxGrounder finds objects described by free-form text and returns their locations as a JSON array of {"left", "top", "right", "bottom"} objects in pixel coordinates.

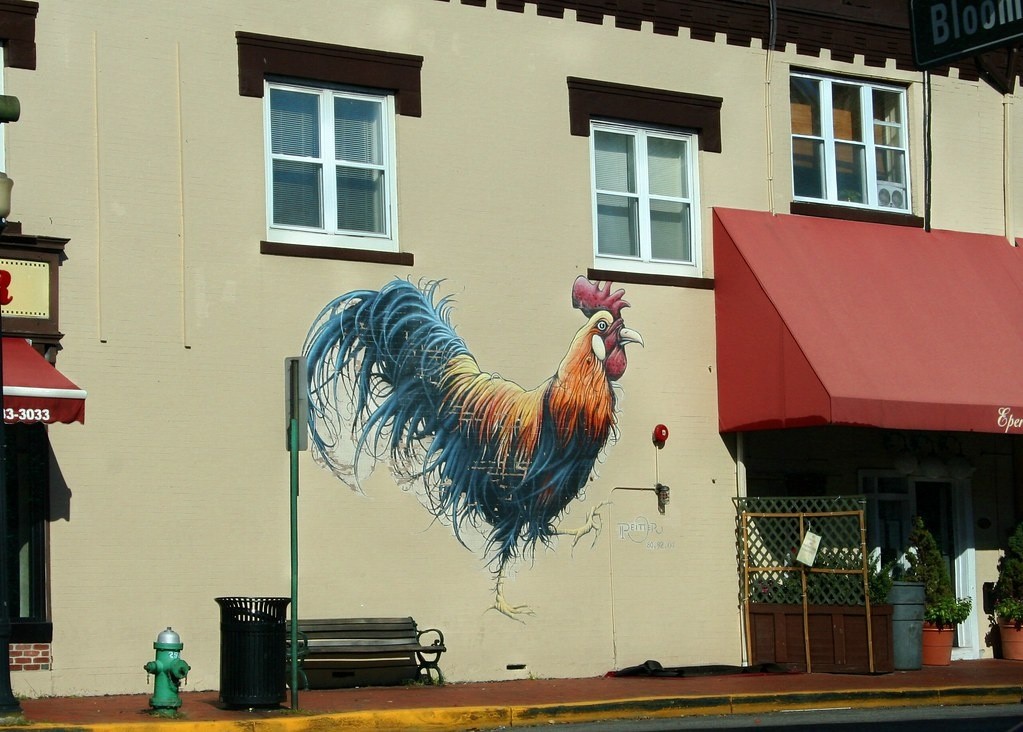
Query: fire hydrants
[{"left": 143, "top": 625, "right": 192, "bottom": 717}]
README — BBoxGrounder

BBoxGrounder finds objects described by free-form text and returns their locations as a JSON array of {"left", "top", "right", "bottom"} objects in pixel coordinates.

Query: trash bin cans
[
  {"left": 887, "top": 580, "right": 926, "bottom": 671},
  {"left": 214, "top": 596, "right": 292, "bottom": 711}
]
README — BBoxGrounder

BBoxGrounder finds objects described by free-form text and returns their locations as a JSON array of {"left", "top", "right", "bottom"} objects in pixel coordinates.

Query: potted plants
[
  {"left": 992, "top": 593, "right": 1023, "bottom": 661},
  {"left": 743, "top": 513, "right": 973, "bottom": 674}
]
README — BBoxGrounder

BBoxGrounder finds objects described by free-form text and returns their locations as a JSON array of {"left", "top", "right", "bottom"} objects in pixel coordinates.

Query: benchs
[{"left": 282, "top": 615, "right": 447, "bottom": 693}]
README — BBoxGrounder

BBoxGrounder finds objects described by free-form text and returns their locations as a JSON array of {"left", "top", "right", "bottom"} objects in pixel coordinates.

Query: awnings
[
  {"left": 712, "top": 206, "right": 1023, "bottom": 434},
  {"left": 2, "top": 337, "right": 87, "bottom": 425}
]
